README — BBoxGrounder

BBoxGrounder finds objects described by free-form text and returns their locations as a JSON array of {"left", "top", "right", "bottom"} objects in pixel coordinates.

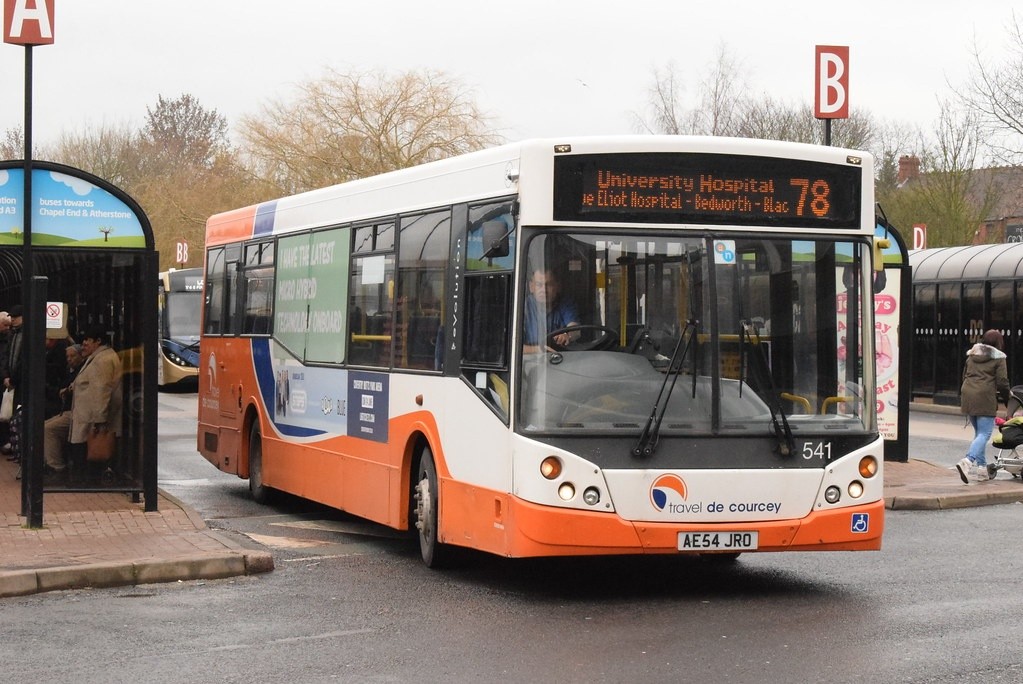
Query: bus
[
  {"left": 195, "top": 136, "right": 891, "bottom": 569},
  {"left": 158, "top": 267, "right": 203, "bottom": 390}
]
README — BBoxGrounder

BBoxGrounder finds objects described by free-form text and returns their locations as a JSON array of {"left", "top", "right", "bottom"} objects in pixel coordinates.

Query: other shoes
[{"left": 43, "top": 471, "right": 66, "bottom": 487}]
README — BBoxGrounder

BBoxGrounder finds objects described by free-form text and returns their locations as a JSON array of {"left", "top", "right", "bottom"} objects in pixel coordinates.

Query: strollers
[{"left": 984, "top": 385, "right": 1023, "bottom": 481}]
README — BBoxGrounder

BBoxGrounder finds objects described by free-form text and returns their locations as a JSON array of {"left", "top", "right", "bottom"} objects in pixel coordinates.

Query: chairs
[
  {"left": 372, "top": 309, "right": 440, "bottom": 363},
  {"left": 242, "top": 307, "right": 272, "bottom": 334}
]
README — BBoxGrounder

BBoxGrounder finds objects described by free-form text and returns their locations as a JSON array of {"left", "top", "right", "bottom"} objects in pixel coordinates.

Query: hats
[
  {"left": 7, "top": 305, "right": 21, "bottom": 316},
  {"left": 45, "top": 328, "right": 69, "bottom": 339}
]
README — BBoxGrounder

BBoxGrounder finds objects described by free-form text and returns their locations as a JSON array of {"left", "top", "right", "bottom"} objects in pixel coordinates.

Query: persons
[
  {"left": 0, "top": 305, "right": 77, "bottom": 463},
  {"left": 956, "top": 330, "right": 1010, "bottom": 484},
  {"left": 993, "top": 406, "right": 1023, "bottom": 444},
  {"left": 522, "top": 267, "right": 581, "bottom": 353},
  {"left": 45, "top": 322, "right": 123, "bottom": 481},
  {"left": 58, "top": 344, "right": 86, "bottom": 412}
]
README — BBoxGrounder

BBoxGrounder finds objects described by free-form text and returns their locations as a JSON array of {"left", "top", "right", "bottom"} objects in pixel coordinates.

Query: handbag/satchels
[
  {"left": 86, "top": 431, "right": 115, "bottom": 461},
  {"left": 0, "top": 387, "right": 15, "bottom": 422}
]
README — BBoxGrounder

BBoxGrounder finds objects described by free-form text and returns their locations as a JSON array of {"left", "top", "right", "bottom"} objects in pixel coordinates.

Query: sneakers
[
  {"left": 957, "top": 458, "right": 971, "bottom": 484},
  {"left": 977, "top": 466, "right": 989, "bottom": 481}
]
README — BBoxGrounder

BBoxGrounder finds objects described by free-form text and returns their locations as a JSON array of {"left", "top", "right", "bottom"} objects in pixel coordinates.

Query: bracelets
[{"left": 565, "top": 331, "right": 571, "bottom": 339}]
